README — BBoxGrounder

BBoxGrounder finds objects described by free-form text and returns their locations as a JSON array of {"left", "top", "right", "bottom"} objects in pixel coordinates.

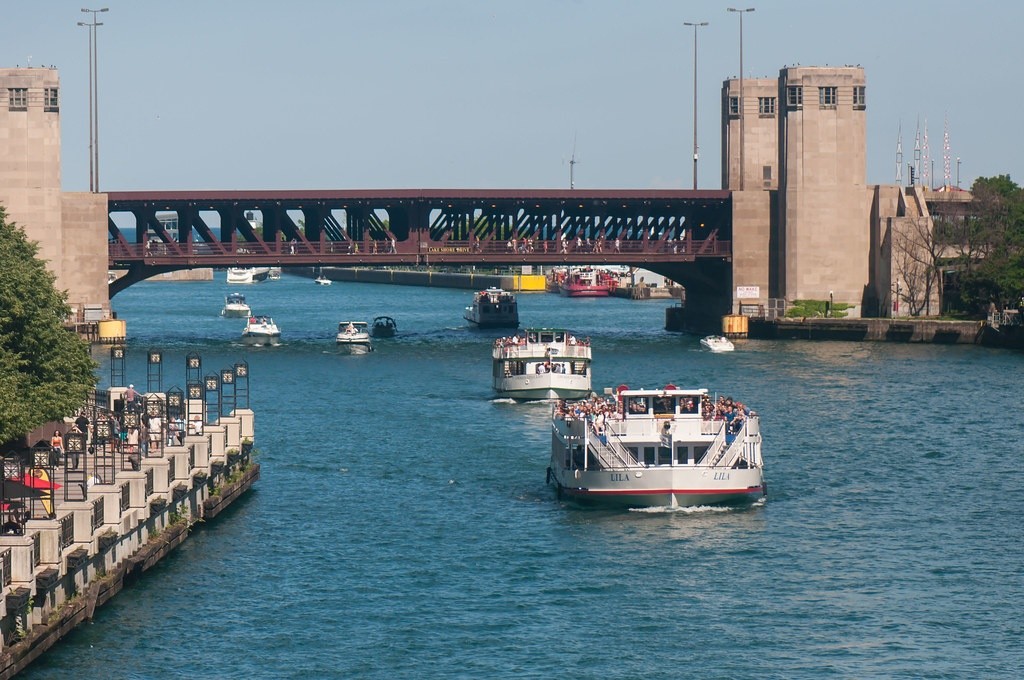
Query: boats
[
  {"left": 241, "top": 315, "right": 282, "bottom": 345},
  {"left": 108, "top": 271, "right": 117, "bottom": 285},
  {"left": 546, "top": 387, "right": 767, "bottom": 510},
  {"left": 226, "top": 267, "right": 271, "bottom": 284},
  {"left": 491, "top": 327, "right": 592, "bottom": 403},
  {"left": 335, "top": 321, "right": 374, "bottom": 355},
  {"left": 268, "top": 267, "right": 281, "bottom": 281},
  {"left": 221, "top": 292, "right": 252, "bottom": 318},
  {"left": 462, "top": 287, "right": 521, "bottom": 331},
  {"left": 545, "top": 265, "right": 619, "bottom": 297},
  {"left": 314, "top": 276, "right": 332, "bottom": 286},
  {"left": 372, "top": 316, "right": 399, "bottom": 338},
  {"left": 699, "top": 334, "right": 736, "bottom": 353}
]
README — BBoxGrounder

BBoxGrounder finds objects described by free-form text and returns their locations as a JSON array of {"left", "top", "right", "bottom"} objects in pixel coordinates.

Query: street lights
[
  {"left": 683, "top": 21, "right": 709, "bottom": 190},
  {"left": 891, "top": 278, "right": 903, "bottom": 317},
  {"left": 907, "top": 162, "right": 910, "bottom": 187},
  {"left": 956, "top": 157, "right": 962, "bottom": 188},
  {"left": 80, "top": 7, "right": 109, "bottom": 193},
  {"left": 931, "top": 159, "right": 934, "bottom": 189},
  {"left": 726, "top": 7, "right": 756, "bottom": 191},
  {"left": 76, "top": 21, "right": 103, "bottom": 193}
]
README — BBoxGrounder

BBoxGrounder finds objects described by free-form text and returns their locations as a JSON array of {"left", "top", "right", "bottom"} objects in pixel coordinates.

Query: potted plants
[{"left": 7, "top": 437, "right": 253, "bottom": 609}]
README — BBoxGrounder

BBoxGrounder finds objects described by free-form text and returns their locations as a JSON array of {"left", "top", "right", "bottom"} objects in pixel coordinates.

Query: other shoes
[{"left": 54, "top": 466, "right": 59, "bottom": 470}]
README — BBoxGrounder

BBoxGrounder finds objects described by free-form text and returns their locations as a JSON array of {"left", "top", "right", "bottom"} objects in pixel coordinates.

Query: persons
[
  {"left": 348, "top": 237, "right": 398, "bottom": 254},
  {"left": 3, "top": 514, "right": 24, "bottom": 534},
  {"left": 473, "top": 233, "right": 685, "bottom": 254},
  {"left": 108, "top": 384, "right": 186, "bottom": 461},
  {"left": 1018, "top": 296, "right": 1024, "bottom": 326},
  {"left": 493, "top": 334, "right": 591, "bottom": 375},
  {"left": 50, "top": 430, "right": 64, "bottom": 471},
  {"left": 280, "top": 237, "right": 299, "bottom": 255},
  {"left": 555, "top": 388, "right": 755, "bottom": 434},
  {"left": 249, "top": 315, "right": 359, "bottom": 335},
  {"left": 145, "top": 240, "right": 159, "bottom": 258},
  {"left": 75, "top": 412, "right": 93, "bottom": 451}
]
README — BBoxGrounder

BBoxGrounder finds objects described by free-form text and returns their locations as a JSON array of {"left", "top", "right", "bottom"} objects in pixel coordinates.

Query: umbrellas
[{"left": 0, "top": 473, "right": 61, "bottom": 520}]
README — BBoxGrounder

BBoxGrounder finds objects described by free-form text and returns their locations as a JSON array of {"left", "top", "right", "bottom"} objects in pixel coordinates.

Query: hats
[
  {"left": 80, "top": 411, "right": 86, "bottom": 415},
  {"left": 598, "top": 409, "right": 604, "bottom": 412},
  {"left": 129, "top": 384, "right": 134, "bottom": 387},
  {"left": 264, "top": 318, "right": 266, "bottom": 320}
]
